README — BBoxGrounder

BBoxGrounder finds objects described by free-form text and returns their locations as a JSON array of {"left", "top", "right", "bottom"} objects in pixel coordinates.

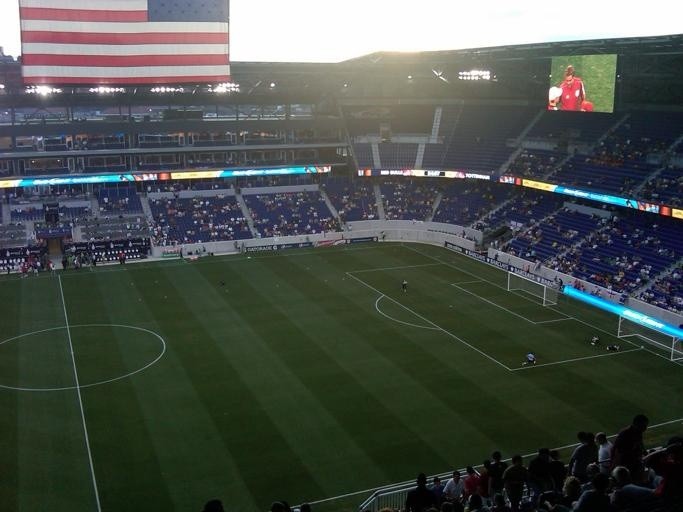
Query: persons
[
  {"left": 549, "top": 85, "right": 564, "bottom": 110},
  {"left": 520, "top": 351, "right": 537, "bottom": 368},
  {"left": 201, "top": 499, "right": 313, "bottom": 512},
  {"left": 590, "top": 335, "right": 600, "bottom": 346},
  {"left": 606, "top": 343, "right": 621, "bottom": 352},
  {"left": 553, "top": 65, "right": 585, "bottom": 111},
  {"left": 580, "top": 100, "right": 594, "bottom": 112}
]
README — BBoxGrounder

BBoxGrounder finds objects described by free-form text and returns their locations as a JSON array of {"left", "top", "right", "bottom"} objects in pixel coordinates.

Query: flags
[{"left": 18, "top": 0, "right": 230, "bottom": 86}]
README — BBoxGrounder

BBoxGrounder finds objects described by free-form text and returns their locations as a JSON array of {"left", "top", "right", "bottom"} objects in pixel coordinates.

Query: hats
[
  {"left": 581, "top": 101, "right": 592, "bottom": 112},
  {"left": 548, "top": 85, "right": 563, "bottom": 100}
]
